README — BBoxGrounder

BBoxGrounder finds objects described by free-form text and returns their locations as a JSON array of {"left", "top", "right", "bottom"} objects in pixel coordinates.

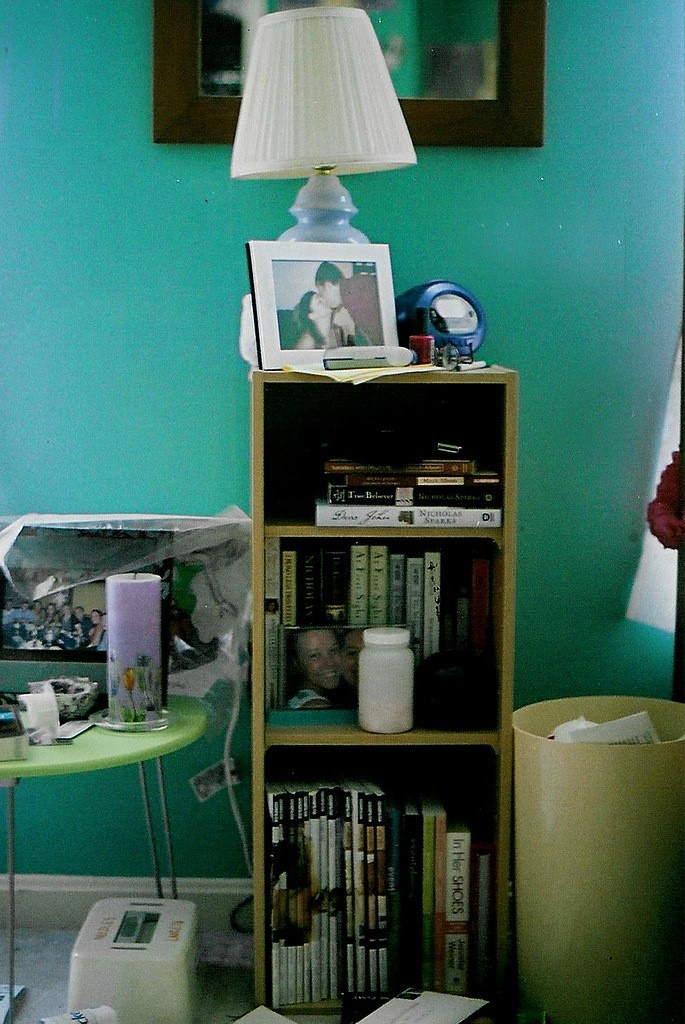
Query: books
[
  {"left": 265, "top": 778, "right": 493, "bottom": 1010},
  {"left": 266, "top": 538, "right": 492, "bottom": 711},
  {"left": 314, "top": 450, "right": 501, "bottom": 528}
]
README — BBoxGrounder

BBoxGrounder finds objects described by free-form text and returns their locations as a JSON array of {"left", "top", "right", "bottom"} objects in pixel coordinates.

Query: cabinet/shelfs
[{"left": 249, "top": 363, "right": 519, "bottom": 1024}]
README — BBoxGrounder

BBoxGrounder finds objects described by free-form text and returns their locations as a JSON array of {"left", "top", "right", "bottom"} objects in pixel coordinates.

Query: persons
[
  {"left": 342, "top": 631, "right": 363, "bottom": 710},
  {"left": 289, "top": 630, "right": 342, "bottom": 709},
  {"left": 294, "top": 261, "right": 385, "bottom": 349},
  {"left": 2, "top": 600, "right": 106, "bottom": 650}
]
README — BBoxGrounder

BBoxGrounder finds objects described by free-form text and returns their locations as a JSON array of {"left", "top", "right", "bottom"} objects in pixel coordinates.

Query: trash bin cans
[{"left": 513, "top": 695, "right": 684, "bottom": 1024}]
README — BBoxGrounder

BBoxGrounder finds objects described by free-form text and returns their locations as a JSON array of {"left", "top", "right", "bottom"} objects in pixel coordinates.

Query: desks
[{"left": 0, "top": 696, "right": 208, "bottom": 1024}]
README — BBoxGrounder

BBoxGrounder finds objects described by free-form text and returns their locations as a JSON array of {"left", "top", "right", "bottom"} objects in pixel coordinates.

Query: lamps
[{"left": 228, "top": 7, "right": 418, "bottom": 369}]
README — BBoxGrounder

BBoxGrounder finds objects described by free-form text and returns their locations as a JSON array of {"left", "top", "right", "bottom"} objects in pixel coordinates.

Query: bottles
[
  {"left": 409, "top": 308, "right": 433, "bottom": 365},
  {"left": 358, "top": 627, "right": 415, "bottom": 733}
]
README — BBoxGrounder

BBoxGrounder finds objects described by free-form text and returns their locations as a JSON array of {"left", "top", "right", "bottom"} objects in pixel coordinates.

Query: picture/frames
[
  {"left": 0, "top": 526, "right": 173, "bottom": 708},
  {"left": 150, "top": 0, "right": 544, "bottom": 147},
  {"left": 245, "top": 240, "right": 399, "bottom": 370},
  {"left": 276, "top": 624, "right": 416, "bottom": 712}
]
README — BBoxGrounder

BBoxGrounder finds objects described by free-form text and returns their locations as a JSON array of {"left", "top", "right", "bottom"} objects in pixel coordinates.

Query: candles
[{"left": 105, "top": 571, "right": 163, "bottom": 729}]
